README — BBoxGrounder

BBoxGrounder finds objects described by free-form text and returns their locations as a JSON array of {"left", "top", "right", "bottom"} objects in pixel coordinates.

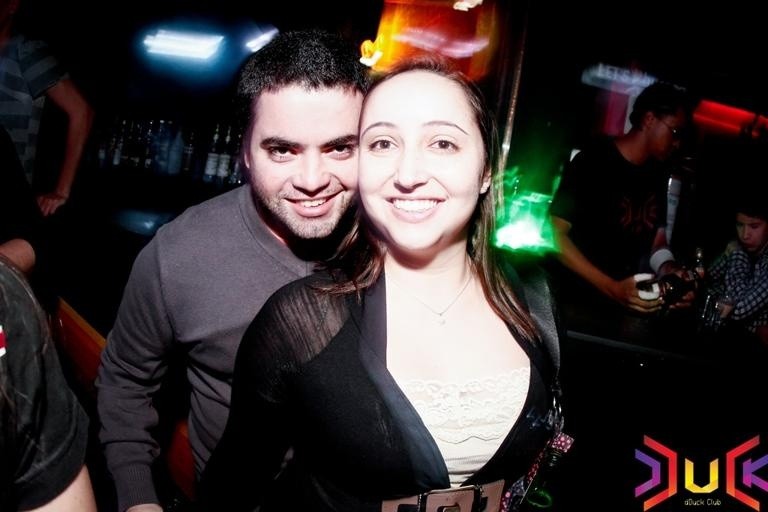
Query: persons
[
  {"left": 194, "top": 61, "right": 561, "bottom": 511},
  {"left": 0, "top": 127, "right": 62, "bottom": 341},
  {"left": 693, "top": 180, "right": 768, "bottom": 364},
  {"left": 93, "top": 28, "right": 380, "bottom": 512},
  {"left": 0, "top": 259, "right": 98, "bottom": 510},
  {"left": 1, "top": 1, "right": 90, "bottom": 216},
  {"left": 542, "top": 80, "right": 698, "bottom": 346}
]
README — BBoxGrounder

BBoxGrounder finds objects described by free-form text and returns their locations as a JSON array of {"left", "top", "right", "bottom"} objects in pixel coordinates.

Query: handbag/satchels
[{"left": 503, "top": 255, "right": 576, "bottom": 507}]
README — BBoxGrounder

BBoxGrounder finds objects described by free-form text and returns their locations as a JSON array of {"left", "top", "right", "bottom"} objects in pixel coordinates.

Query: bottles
[
  {"left": 637, "top": 267, "right": 703, "bottom": 306},
  {"left": 98, "top": 118, "right": 245, "bottom": 186}
]
